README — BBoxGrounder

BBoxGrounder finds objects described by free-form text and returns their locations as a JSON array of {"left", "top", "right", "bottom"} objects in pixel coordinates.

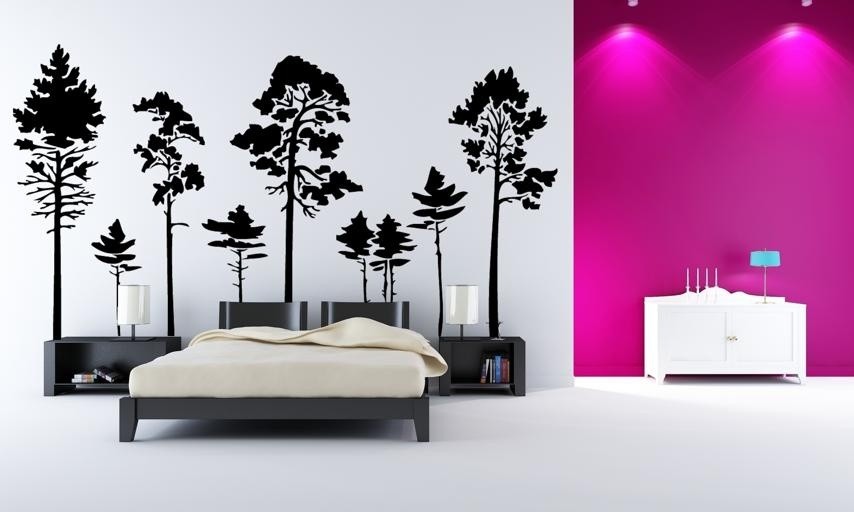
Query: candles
[{"left": 685, "top": 267, "right": 718, "bottom": 287}]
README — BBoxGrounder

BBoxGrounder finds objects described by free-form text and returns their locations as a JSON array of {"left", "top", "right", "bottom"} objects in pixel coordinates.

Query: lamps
[
  {"left": 750, "top": 248, "right": 781, "bottom": 305},
  {"left": 443, "top": 285, "right": 483, "bottom": 342},
  {"left": 117, "top": 284, "right": 155, "bottom": 341}
]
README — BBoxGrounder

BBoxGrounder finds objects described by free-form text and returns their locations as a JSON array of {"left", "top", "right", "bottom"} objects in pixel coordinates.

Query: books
[
  {"left": 71, "top": 373, "right": 97, "bottom": 384},
  {"left": 93, "top": 365, "right": 121, "bottom": 383},
  {"left": 480, "top": 355, "right": 511, "bottom": 384}
]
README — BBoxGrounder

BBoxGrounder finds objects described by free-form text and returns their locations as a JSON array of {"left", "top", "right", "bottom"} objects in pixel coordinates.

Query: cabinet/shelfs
[{"left": 644, "top": 287, "right": 807, "bottom": 387}]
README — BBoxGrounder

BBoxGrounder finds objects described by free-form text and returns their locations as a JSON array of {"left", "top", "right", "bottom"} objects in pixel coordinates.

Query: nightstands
[
  {"left": 44, "top": 336, "right": 181, "bottom": 399},
  {"left": 440, "top": 335, "right": 527, "bottom": 396}
]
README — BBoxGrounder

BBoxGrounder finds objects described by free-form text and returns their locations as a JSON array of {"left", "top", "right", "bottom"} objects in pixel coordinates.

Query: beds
[{"left": 119, "top": 301, "right": 430, "bottom": 442}]
